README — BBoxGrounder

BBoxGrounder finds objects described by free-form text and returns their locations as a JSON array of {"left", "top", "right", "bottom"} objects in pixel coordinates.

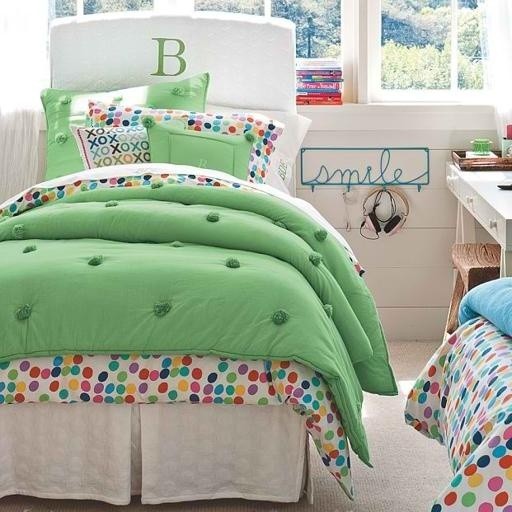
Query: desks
[{"left": 446, "top": 161, "right": 512, "bottom": 278}]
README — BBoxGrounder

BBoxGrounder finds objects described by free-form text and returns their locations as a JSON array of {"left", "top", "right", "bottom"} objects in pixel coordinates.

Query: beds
[{"left": 0, "top": 9, "right": 313, "bottom": 505}]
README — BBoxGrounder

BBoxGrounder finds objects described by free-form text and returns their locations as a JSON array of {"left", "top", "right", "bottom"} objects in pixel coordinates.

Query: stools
[{"left": 448, "top": 243, "right": 501, "bottom": 333}]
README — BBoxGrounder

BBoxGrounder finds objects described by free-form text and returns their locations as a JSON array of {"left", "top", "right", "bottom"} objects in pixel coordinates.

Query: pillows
[{"left": 40, "top": 73, "right": 312, "bottom": 194}]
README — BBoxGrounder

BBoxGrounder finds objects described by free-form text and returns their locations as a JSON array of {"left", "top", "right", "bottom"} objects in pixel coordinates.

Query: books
[{"left": 296, "top": 56, "right": 345, "bottom": 106}]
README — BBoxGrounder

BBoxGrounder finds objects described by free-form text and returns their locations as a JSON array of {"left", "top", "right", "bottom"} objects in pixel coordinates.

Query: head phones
[{"left": 361, "top": 186, "right": 409, "bottom": 237}]
